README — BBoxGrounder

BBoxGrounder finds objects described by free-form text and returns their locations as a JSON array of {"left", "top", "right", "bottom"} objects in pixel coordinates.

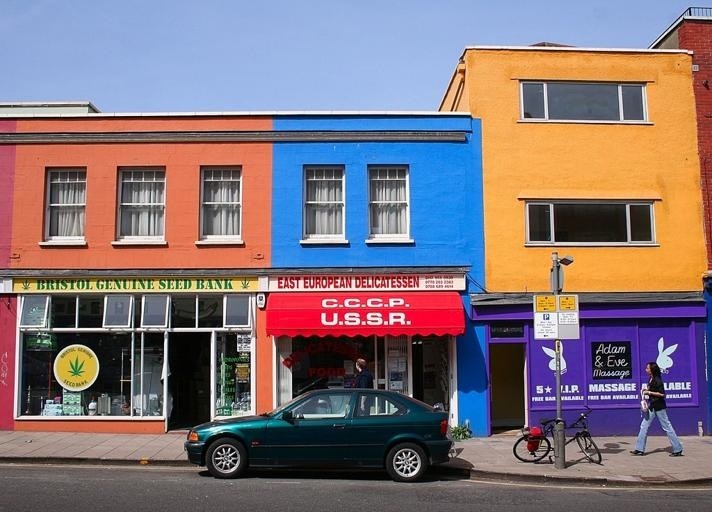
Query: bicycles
[{"left": 513, "top": 405, "right": 602, "bottom": 464}]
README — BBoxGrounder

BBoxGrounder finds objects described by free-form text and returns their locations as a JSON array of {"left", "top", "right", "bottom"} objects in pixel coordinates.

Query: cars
[{"left": 183, "top": 388, "right": 455, "bottom": 483}]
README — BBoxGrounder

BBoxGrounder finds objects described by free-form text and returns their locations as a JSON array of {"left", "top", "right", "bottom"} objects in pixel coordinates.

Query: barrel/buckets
[{"left": 88, "top": 400, "right": 98, "bottom": 416}]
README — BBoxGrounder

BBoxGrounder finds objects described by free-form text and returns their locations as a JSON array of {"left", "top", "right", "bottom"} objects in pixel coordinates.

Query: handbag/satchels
[{"left": 640, "top": 389, "right": 649, "bottom": 412}]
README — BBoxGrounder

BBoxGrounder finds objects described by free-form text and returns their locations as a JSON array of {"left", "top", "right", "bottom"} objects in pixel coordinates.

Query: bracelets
[{"left": 648, "top": 391, "right": 651, "bottom": 395}]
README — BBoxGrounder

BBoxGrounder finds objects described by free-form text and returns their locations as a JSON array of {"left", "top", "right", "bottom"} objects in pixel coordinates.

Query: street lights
[{"left": 552, "top": 252, "right": 573, "bottom": 469}]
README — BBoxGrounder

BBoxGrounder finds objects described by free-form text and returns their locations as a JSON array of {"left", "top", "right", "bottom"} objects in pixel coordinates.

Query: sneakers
[
  {"left": 629, "top": 449, "right": 644, "bottom": 455},
  {"left": 668, "top": 449, "right": 682, "bottom": 456}
]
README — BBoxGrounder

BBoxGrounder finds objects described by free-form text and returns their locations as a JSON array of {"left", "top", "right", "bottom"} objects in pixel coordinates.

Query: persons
[
  {"left": 628, "top": 361, "right": 683, "bottom": 456},
  {"left": 352, "top": 358, "right": 373, "bottom": 415}
]
